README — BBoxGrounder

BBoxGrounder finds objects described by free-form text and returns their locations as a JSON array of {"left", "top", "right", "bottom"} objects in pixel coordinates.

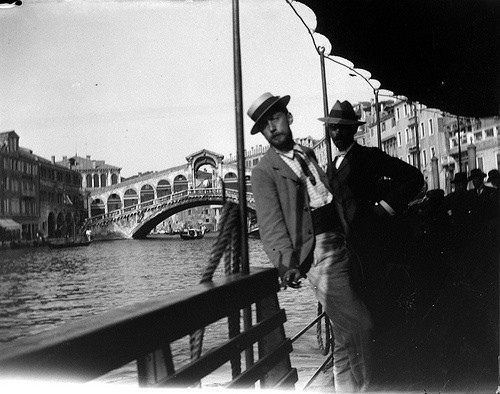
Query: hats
[
  {"left": 469, "top": 168, "right": 486, "bottom": 181},
  {"left": 318, "top": 100, "right": 366, "bottom": 125},
  {"left": 450, "top": 172, "right": 469, "bottom": 184},
  {"left": 247, "top": 93, "right": 290, "bottom": 135},
  {"left": 486, "top": 169, "right": 500, "bottom": 183}
]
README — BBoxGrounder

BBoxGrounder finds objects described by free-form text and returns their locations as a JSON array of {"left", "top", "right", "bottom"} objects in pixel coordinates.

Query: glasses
[{"left": 330, "top": 123, "right": 353, "bottom": 129}]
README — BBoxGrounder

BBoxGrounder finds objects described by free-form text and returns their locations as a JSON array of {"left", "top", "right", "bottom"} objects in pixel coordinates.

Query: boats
[
  {"left": 180, "top": 226, "right": 206, "bottom": 239},
  {"left": 46, "top": 230, "right": 93, "bottom": 246}
]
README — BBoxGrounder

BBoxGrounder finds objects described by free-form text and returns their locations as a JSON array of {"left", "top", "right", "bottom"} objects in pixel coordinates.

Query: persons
[
  {"left": 425, "top": 169, "right": 500, "bottom": 220},
  {"left": 247, "top": 92, "right": 375, "bottom": 394},
  {"left": 318, "top": 100, "right": 424, "bottom": 281}
]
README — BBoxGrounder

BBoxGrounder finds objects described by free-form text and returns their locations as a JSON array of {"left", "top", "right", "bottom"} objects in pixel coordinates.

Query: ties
[{"left": 294, "top": 152, "right": 317, "bottom": 186}]
